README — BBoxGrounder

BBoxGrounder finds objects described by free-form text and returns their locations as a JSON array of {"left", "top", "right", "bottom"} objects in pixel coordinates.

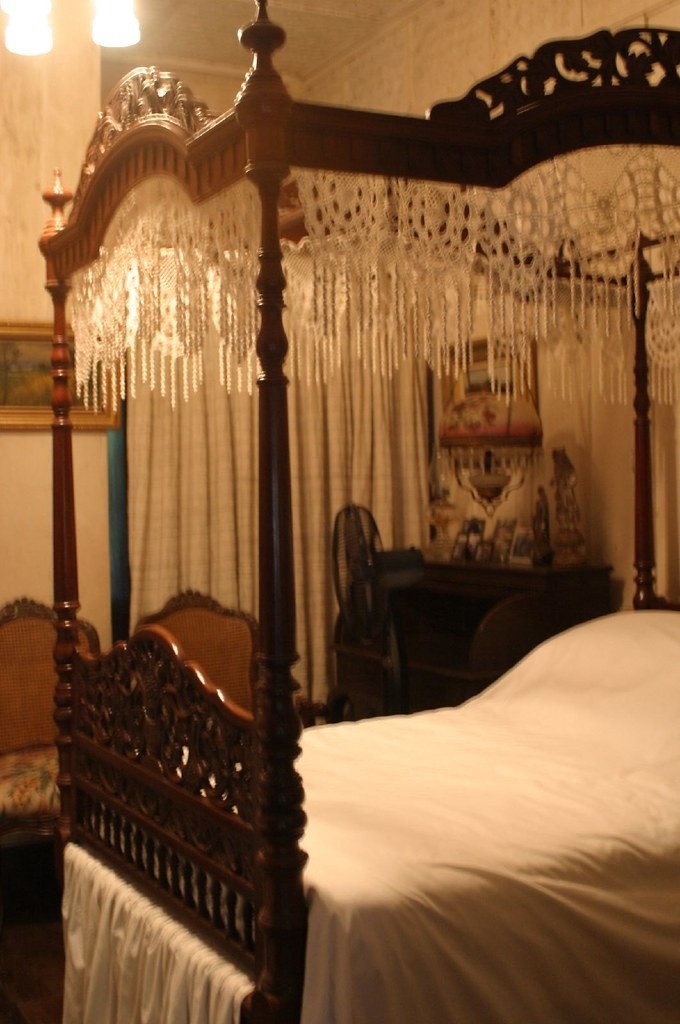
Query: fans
[{"left": 331, "top": 502, "right": 402, "bottom": 716}]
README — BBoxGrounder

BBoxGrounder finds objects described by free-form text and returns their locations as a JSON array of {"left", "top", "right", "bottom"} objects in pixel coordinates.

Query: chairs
[
  {"left": 0, "top": 598, "right": 99, "bottom": 915},
  {"left": 136, "top": 588, "right": 305, "bottom": 736}
]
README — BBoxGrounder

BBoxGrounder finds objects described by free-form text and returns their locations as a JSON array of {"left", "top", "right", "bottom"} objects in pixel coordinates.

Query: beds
[{"left": 37, "top": 0, "right": 680, "bottom": 1024}]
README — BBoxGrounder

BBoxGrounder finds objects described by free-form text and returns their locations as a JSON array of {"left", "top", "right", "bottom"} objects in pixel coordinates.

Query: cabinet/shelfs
[{"left": 330, "top": 548, "right": 609, "bottom": 727}]
